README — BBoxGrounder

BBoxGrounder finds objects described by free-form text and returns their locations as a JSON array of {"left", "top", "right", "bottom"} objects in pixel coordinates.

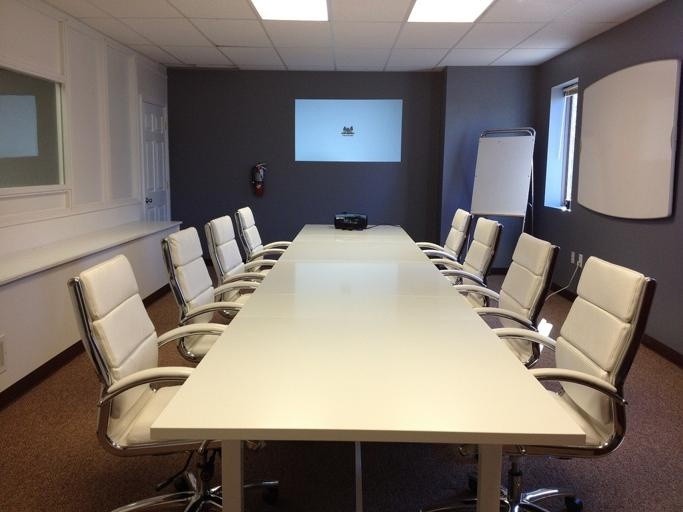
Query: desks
[{"left": 150, "top": 223, "right": 588, "bottom": 508}]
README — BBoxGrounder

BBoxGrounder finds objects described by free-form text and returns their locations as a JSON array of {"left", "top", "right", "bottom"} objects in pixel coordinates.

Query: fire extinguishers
[{"left": 253, "top": 162, "right": 268, "bottom": 197}]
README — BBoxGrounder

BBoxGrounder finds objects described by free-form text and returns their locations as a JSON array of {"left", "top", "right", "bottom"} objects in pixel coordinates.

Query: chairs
[
  {"left": 159, "top": 208, "right": 294, "bottom": 364},
  {"left": 67, "top": 254, "right": 281, "bottom": 511},
  {"left": 442, "top": 254, "right": 655, "bottom": 511},
  {"left": 413, "top": 207, "right": 561, "bottom": 366}
]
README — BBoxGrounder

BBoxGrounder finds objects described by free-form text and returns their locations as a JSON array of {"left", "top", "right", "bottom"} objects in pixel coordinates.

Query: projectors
[{"left": 335, "top": 213, "right": 367, "bottom": 230}]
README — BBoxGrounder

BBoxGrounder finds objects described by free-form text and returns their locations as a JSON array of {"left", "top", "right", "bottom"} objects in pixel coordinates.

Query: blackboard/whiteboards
[{"left": 470, "top": 135, "right": 535, "bottom": 218}]
